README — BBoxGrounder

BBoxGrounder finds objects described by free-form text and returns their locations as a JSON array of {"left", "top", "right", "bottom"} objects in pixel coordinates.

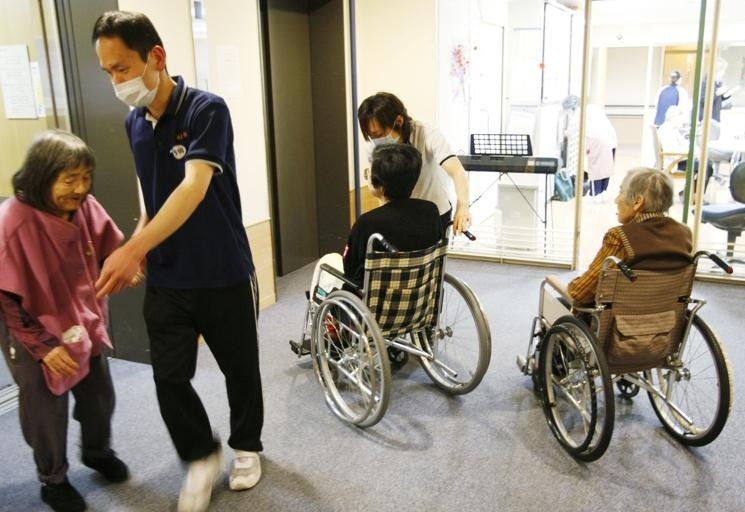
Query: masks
[
  {"left": 113, "top": 51, "right": 158, "bottom": 109},
  {"left": 372, "top": 120, "right": 400, "bottom": 146}
]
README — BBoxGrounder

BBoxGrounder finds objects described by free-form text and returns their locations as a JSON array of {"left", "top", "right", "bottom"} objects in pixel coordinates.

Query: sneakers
[
  {"left": 41, "top": 474, "right": 86, "bottom": 512},
  {"left": 229, "top": 449, "right": 262, "bottom": 491},
  {"left": 178, "top": 447, "right": 226, "bottom": 512},
  {"left": 82, "top": 449, "right": 127, "bottom": 482}
]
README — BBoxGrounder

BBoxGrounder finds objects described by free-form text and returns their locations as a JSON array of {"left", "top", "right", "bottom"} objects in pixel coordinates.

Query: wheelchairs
[
  {"left": 518, "top": 247, "right": 735, "bottom": 466},
  {"left": 290, "top": 220, "right": 489, "bottom": 428}
]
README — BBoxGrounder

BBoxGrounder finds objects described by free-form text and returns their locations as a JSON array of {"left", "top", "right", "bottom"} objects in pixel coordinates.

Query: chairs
[
  {"left": 652, "top": 123, "right": 701, "bottom": 181},
  {"left": 691, "top": 161, "right": 745, "bottom": 273}
]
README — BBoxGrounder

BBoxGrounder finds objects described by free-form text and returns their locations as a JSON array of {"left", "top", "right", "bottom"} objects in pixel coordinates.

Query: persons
[
  {"left": 527, "top": 165, "right": 694, "bottom": 377},
  {"left": 680, "top": 56, "right": 741, "bottom": 221},
  {"left": 0, "top": 128, "right": 142, "bottom": 511},
  {"left": 652, "top": 69, "right": 687, "bottom": 129},
  {"left": 562, "top": 93, "right": 618, "bottom": 197},
  {"left": 355, "top": 91, "right": 472, "bottom": 247},
  {"left": 92, "top": 11, "right": 265, "bottom": 510},
  {"left": 300, "top": 142, "right": 445, "bottom": 350}
]
